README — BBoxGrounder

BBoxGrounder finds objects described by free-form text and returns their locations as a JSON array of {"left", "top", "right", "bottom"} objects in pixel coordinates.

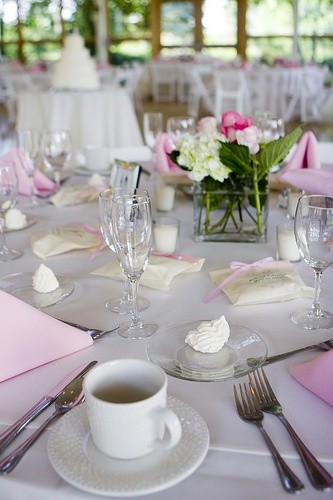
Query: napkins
[
  {"left": 291, "top": 347, "right": 333, "bottom": 405},
  {"left": 1, "top": 149, "right": 59, "bottom": 199},
  {"left": 283, "top": 130, "right": 322, "bottom": 170},
  {"left": 154, "top": 133, "right": 184, "bottom": 174},
  {"left": 0, "top": 290, "right": 94, "bottom": 383}
]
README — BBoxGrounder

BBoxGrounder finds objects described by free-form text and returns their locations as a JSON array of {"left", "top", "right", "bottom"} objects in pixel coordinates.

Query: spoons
[{"left": 0, "top": 376, "right": 86, "bottom": 476}]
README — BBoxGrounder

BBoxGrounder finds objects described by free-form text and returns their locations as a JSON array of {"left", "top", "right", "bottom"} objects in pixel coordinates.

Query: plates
[
  {"left": 75, "top": 163, "right": 111, "bottom": 177},
  {"left": 47, "top": 395, "right": 210, "bottom": 496},
  {"left": 0, "top": 271, "right": 75, "bottom": 310},
  {"left": 146, "top": 319, "right": 269, "bottom": 383},
  {"left": 2, "top": 212, "right": 38, "bottom": 233}
]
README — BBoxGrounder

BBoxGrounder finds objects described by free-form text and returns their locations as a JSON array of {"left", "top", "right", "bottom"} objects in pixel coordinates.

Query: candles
[
  {"left": 287, "top": 193, "right": 309, "bottom": 217},
  {"left": 156, "top": 186, "right": 174, "bottom": 210},
  {"left": 278, "top": 229, "right": 302, "bottom": 261},
  {"left": 154, "top": 225, "right": 177, "bottom": 254}
]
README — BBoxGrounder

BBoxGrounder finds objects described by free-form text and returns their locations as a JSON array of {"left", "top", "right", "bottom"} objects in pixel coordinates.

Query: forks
[
  {"left": 246, "top": 337, "right": 333, "bottom": 366},
  {"left": 233, "top": 382, "right": 306, "bottom": 495},
  {"left": 246, "top": 366, "right": 333, "bottom": 492},
  {"left": 55, "top": 317, "right": 121, "bottom": 340}
]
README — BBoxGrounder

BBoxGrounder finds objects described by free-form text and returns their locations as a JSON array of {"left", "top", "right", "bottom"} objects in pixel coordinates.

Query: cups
[
  {"left": 83, "top": 358, "right": 183, "bottom": 459},
  {"left": 154, "top": 181, "right": 178, "bottom": 214},
  {"left": 250, "top": 108, "right": 286, "bottom": 142},
  {"left": 275, "top": 220, "right": 303, "bottom": 263},
  {"left": 286, "top": 186, "right": 311, "bottom": 221},
  {"left": 167, "top": 116, "right": 197, "bottom": 150},
  {"left": 83, "top": 143, "right": 111, "bottom": 170},
  {"left": 152, "top": 216, "right": 181, "bottom": 257}
]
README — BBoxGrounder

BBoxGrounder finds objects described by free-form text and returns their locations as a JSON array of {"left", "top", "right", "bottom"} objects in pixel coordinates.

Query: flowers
[{"left": 166, "top": 111, "right": 306, "bottom": 234}]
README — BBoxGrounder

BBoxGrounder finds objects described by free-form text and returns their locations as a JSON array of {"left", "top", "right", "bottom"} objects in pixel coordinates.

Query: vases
[{"left": 193, "top": 170, "right": 275, "bottom": 242}]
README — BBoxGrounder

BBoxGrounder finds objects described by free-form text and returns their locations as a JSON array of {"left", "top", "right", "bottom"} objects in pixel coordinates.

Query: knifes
[{"left": 0, "top": 357, "right": 95, "bottom": 454}]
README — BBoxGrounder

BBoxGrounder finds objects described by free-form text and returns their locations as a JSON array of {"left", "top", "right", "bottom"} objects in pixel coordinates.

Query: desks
[
  {"left": 0, "top": 141, "right": 333, "bottom": 500},
  {"left": 15, "top": 88, "right": 143, "bottom": 155}
]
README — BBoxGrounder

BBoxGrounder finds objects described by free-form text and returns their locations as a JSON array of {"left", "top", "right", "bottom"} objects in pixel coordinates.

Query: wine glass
[
  {"left": 290, "top": 194, "right": 333, "bottom": 331},
  {"left": 14, "top": 127, "right": 72, "bottom": 210},
  {"left": 0, "top": 160, "right": 25, "bottom": 261},
  {"left": 142, "top": 111, "right": 164, "bottom": 182},
  {"left": 98, "top": 188, "right": 160, "bottom": 341}
]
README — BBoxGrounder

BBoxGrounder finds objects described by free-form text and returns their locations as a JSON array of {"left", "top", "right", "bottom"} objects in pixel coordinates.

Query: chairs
[
  {"left": 144, "top": 60, "right": 333, "bottom": 128},
  {"left": 0, "top": 60, "right": 141, "bottom": 128}
]
